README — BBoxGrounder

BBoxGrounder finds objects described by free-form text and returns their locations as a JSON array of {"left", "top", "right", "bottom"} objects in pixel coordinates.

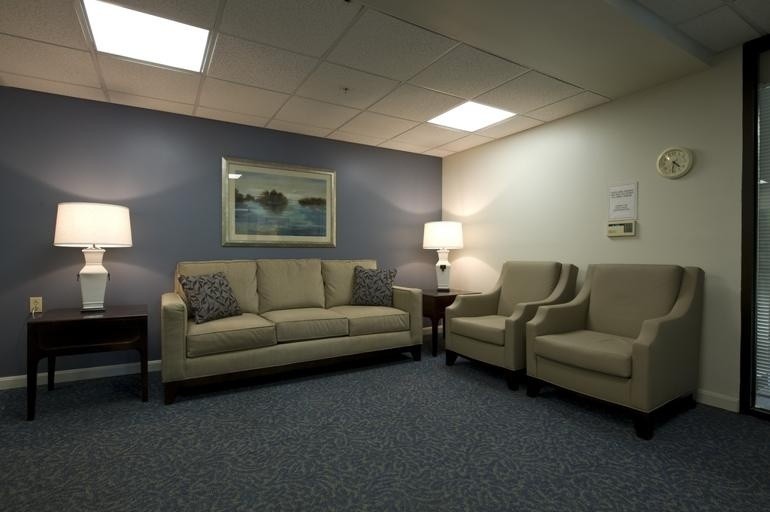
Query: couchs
[{"left": 161, "top": 259, "right": 424, "bottom": 406}]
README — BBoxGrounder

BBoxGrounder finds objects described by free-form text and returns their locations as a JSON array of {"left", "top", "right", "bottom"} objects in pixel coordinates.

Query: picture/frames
[{"left": 222, "top": 155, "right": 337, "bottom": 249}]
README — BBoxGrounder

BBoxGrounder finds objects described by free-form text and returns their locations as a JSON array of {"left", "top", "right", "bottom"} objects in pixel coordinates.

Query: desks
[
  {"left": 416, "top": 287, "right": 481, "bottom": 358},
  {"left": 26, "top": 305, "right": 150, "bottom": 422}
]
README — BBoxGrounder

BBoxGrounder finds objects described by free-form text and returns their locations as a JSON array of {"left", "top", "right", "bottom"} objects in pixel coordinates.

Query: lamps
[
  {"left": 423, "top": 220, "right": 464, "bottom": 294},
  {"left": 54, "top": 200, "right": 132, "bottom": 313}
]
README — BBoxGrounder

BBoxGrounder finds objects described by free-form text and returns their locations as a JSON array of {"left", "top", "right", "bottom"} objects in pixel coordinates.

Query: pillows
[
  {"left": 350, "top": 265, "right": 397, "bottom": 308},
  {"left": 176, "top": 272, "right": 242, "bottom": 324}
]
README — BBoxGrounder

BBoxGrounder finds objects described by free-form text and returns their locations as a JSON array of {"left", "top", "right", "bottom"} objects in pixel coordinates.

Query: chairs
[
  {"left": 526, "top": 263, "right": 705, "bottom": 439},
  {"left": 445, "top": 259, "right": 579, "bottom": 391}
]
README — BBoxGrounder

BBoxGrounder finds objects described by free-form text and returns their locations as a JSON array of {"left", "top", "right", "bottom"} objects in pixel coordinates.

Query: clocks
[{"left": 656, "top": 146, "right": 692, "bottom": 180}]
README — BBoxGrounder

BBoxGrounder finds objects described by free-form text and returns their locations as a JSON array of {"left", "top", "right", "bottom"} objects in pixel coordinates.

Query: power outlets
[{"left": 30, "top": 297, "right": 42, "bottom": 313}]
